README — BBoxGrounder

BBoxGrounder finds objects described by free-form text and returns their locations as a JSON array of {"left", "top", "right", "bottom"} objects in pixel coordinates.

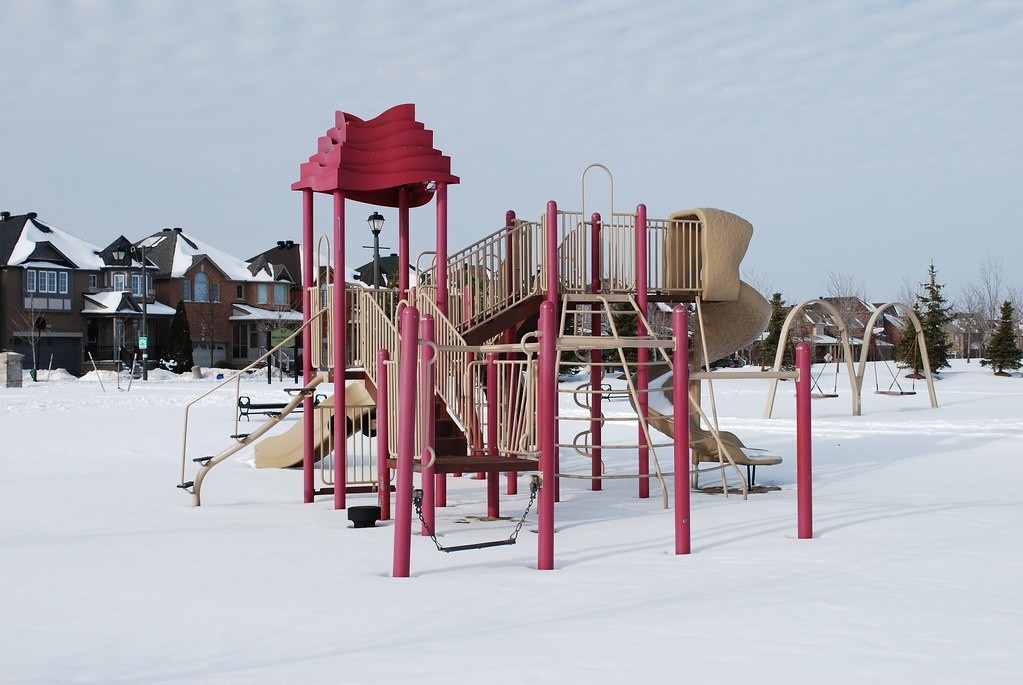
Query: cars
[{"left": 702, "top": 358, "right": 741, "bottom": 372}]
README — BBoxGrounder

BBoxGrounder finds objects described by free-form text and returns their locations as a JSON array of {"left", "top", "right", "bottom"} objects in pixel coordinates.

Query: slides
[{"left": 171, "top": 206, "right": 784, "bottom": 496}]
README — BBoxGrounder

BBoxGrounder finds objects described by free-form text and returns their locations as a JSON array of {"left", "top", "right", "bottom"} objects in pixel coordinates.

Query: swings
[
  {"left": 870, "top": 328, "right": 921, "bottom": 396},
  {"left": 411, "top": 474, "right": 543, "bottom": 553},
  {"left": 787, "top": 328, "right": 845, "bottom": 399}
]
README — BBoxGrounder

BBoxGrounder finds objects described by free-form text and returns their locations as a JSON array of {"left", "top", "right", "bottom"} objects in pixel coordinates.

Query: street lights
[{"left": 362, "top": 210, "right": 391, "bottom": 292}]
